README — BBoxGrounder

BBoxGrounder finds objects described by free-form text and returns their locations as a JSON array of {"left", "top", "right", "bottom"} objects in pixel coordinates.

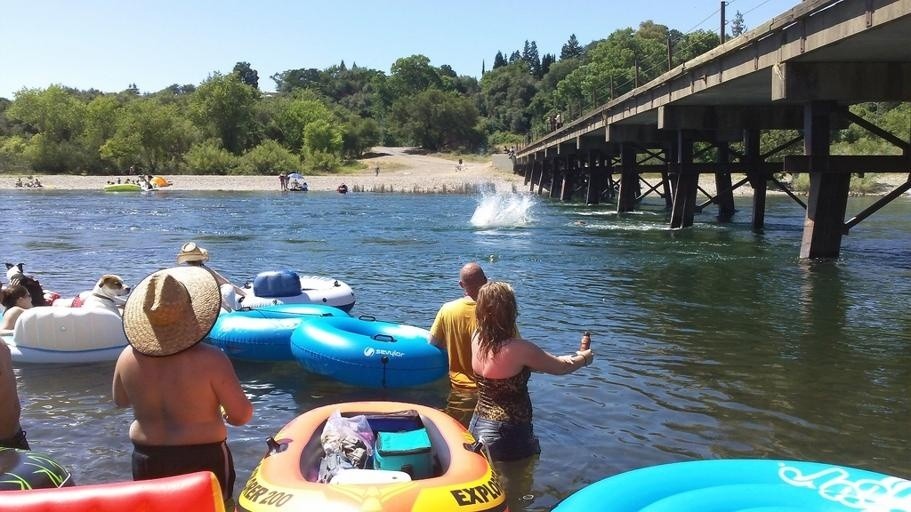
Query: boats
[
  {"left": 105, "top": 184, "right": 142, "bottom": 192},
  {"left": 550, "top": 456, "right": 911, "bottom": 512},
  {"left": 150, "top": 177, "right": 170, "bottom": 187},
  {"left": 234, "top": 399, "right": 514, "bottom": 512},
  {"left": 286, "top": 172, "right": 309, "bottom": 191},
  {"left": 1, "top": 467, "right": 228, "bottom": 510},
  {"left": 1, "top": 286, "right": 133, "bottom": 365}
]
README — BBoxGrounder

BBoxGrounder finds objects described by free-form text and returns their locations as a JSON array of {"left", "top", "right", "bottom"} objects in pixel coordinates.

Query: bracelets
[{"left": 579, "top": 353, "right": 588, "bottom": 367}]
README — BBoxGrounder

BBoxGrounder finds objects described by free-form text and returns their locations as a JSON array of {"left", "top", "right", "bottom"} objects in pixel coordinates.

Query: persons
[
  {"left": 23, "top": 180, "right": 32, "bottom": 188},
  {"left": 291, "top": 178, "right": 300, "bottom": 189},
  {"left": 301, "top": 182, "right": 308, "bottom": 191},
  {"left": 465, "top": 282, "right": 595, "bottom": 462},
  {"left": 504, "top": 145, "right": 510, "bottom": 154},
  {"left": 106, "top": 175, "right": 153, "bottom": 189},
  {"left": 554, "top": 112, "right": 562, "bottom": 130},
  {"left": 109, "top": 265, "right": 253, "bottom": 512},
  {"left": 545, "top": 116, "right": 553, "bottom": 133},
  {"left": 509, "top": 145, "right": 515, "bottom": 159},
  {"left": 0, "top": 337, "right": 32, "bottom": 452},
  {"left": 174, "top": 241, "right": 209, "bottom": 267},
  {"left": 14, "top": 178, "right": 21, "bottom": 187},
  {"left": 491, "top": 453, "right": 572, "bottom": 512},
  {"left": 33, "top": 178, "right": 40, "bottom": 188},
  {"left": 0, "top": 284, "right": 34, "bottom": 330},
  {"left": 425, "top": 262, "right": 522, "bottom": 390},
  {"left": 278, "top": 174, "right": 287, "bottom": 191},
  {"left": 550, "top": 113, "right": 556, "bottom": 132},
  {"left": 375, "top": 166, "right": 380, "bottom": 176}
]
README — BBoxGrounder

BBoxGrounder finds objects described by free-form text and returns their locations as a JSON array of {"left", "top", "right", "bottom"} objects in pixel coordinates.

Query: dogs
[
  {"left": 50, "top": 274, "right": 132, "bottom": 318},
  {"left": 5, "top": 263, "right": 47, "bottom": 307}
]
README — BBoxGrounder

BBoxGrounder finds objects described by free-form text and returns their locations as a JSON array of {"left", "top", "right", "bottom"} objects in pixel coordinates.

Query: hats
[
  {"left": 177, "top": 242, "right": 208, "bottom": 264},
  {"left": 122, "top": 266, "right": 222, "bottom": 356}
]
